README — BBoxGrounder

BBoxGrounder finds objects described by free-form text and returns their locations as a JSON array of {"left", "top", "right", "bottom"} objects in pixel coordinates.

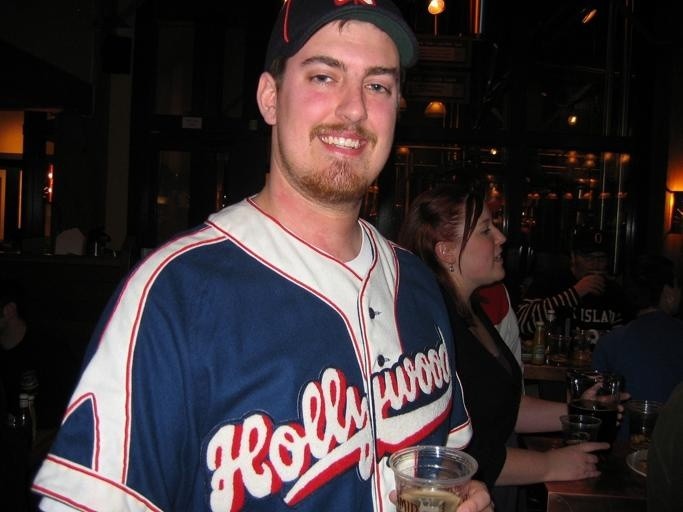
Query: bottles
[
  {"left": 532, "top": 311, "right": 592, "bottom": 365},
  {"left": 12, "top": 395, "right": 36, "bottom": 453}
]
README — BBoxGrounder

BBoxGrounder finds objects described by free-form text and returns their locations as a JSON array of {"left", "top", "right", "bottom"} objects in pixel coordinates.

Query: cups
[
  {"left": 387, "top": 446, "right": 479, "bottom": 512},
  {"left": 558, "top": 400, "right": 664, "bottom": 451}
]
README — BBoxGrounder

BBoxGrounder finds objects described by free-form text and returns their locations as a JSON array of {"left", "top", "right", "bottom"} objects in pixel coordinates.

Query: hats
[{"left": 263, "top": 0, "right": 419, "bottom": 71}]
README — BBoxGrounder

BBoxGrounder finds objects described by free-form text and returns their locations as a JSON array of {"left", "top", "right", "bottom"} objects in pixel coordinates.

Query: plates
[{"left": 625, "top": 451, "right": 648, "bottom": 485}]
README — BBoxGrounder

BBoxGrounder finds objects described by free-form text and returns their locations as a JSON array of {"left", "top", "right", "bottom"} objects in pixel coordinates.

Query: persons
[
  {"left": 397, "top": 181, "right": 683, "bottom": 512},
  {"left": 30, "top": 0, "right": 497, "bottom": 512},
  {"left": 0, "top": 279, "right": 69, "bottom": 430}
]
